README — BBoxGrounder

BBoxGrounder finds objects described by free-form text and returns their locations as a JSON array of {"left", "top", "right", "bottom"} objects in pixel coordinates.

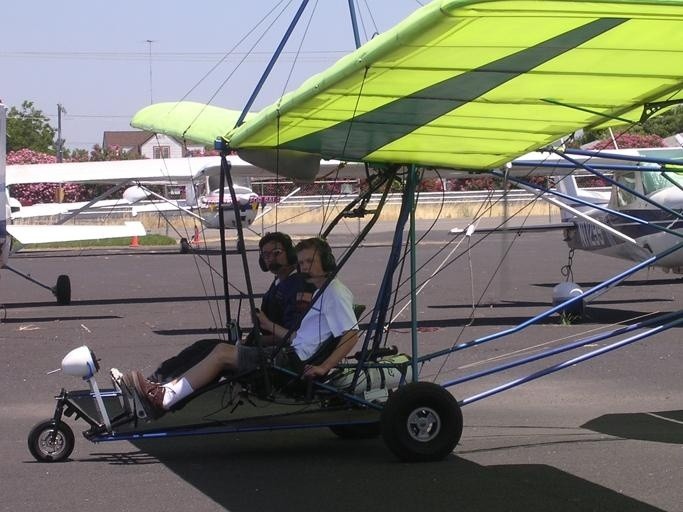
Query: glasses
[{"left": 262, "top": 248, "right": 285, "bottom": 258}]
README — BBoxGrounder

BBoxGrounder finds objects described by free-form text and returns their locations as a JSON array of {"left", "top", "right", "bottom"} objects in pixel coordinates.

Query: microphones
[
  {"left": 300, "top": 272, "right": 333, "bottom": 279},
  {"left": 272, "top": 263, "right": 292, "bottom": 268}
]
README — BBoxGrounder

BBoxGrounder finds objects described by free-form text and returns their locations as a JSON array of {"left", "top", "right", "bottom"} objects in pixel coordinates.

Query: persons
[
  {"left": 132, "top": 236, "right": 357, "bottom": 419},
  {"left": 108, "top": 231, "right": 319, "bottom": 416}
]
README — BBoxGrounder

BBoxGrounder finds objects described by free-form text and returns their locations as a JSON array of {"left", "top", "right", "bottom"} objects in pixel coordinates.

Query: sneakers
[{"left": 110, "top": 368, "right": 170, "bottom": 421}]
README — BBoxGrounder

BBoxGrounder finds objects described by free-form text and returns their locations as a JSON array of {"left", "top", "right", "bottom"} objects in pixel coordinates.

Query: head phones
[
  {"left": 259, "top": 231, "right": 297, "bottom": 272},
  {"left": 296, "top": 238, "right": 336, "bottom": 273}
]
